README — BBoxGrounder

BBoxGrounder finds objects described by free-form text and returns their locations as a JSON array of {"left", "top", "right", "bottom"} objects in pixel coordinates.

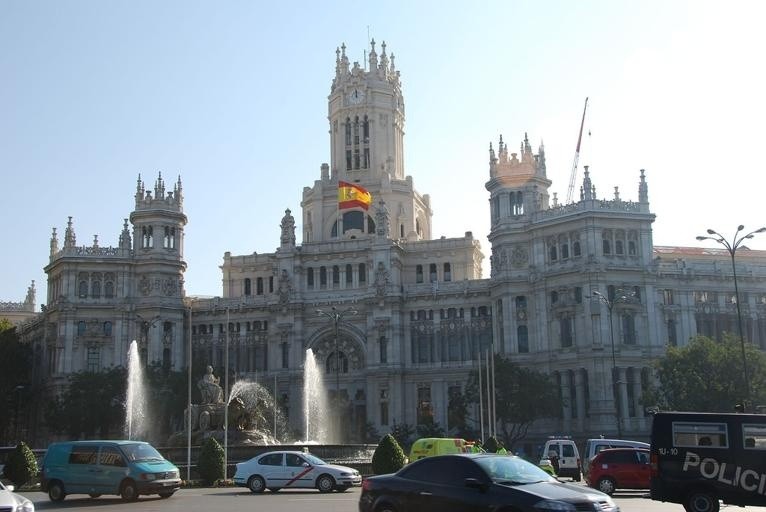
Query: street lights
[
  {"left": 696, "top": 223, "right": 765, "bottom": 408},
  {"left": 129, "top": 313, "right": 162, "bottom": 441},
  {"left": 586, "top": 289, "right": 636, "bottom": 438},
  {"left": 314, "top": 305, "right": 357, "bottom": 441}
]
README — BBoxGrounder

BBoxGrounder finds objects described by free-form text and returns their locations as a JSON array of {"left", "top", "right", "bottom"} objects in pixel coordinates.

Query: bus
[{"left": 0, "top": 447, "right": 45, "bottom": 483}]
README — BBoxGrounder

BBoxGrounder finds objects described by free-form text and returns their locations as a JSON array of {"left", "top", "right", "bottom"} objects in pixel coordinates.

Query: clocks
[{"left": 348, "top": 87, "right": 365, "bottom": 105}]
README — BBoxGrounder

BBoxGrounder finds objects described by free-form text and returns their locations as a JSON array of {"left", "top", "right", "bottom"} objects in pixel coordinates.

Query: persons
[
  {"left": 201, "top": 364, "right": 221, "bottom": 403},
  {"left": 495, "top": 438, "right": 506, "bottom": 455},
  {"left": 472, "top": 438, "right": 483, "bottom": 454}
]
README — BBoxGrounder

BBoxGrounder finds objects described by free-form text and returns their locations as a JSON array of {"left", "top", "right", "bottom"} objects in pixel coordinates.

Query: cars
[
  {"left": 358, "top": 452, "right": 621, "bottom": 512},
  {"left": 583, "top": 447, "right": 650, "bottom": 496},
  {"left": 0, "top": 481, "right": 34, "bottom": 512},
  {"left": 232, "top": 446, "right": 363, "bottom": 494}
]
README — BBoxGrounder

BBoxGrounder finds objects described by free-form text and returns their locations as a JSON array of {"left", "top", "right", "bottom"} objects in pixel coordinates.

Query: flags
[{"left": 337, "top": 180, "right": 371, "bottom": 211}]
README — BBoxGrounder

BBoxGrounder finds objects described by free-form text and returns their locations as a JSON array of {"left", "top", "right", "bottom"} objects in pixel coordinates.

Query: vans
[
  {"left": 39, "top": 440, "right": 184, "bottom": 502},
  {"left": 648, "top": 405, "right": 766, "bottom": 512},
  {"left": 539, "top": 435, "right": 582, "bottom": 482},
  {"left": 408, "top": 437, "right": 487, "bottom": 464},
  {"left": 582, "top": 435, "right": 650, "bottom": 472}
]
